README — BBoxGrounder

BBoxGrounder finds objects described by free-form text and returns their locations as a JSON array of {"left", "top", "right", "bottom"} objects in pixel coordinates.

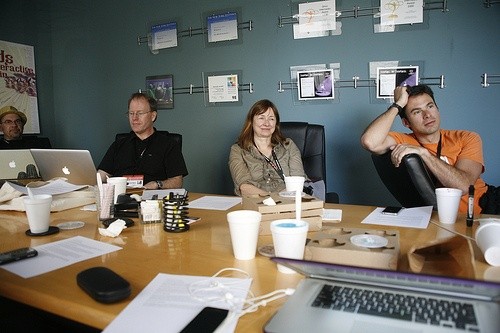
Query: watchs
[{"left": 156, "top": 180, "right": 163, "bottom": 189}]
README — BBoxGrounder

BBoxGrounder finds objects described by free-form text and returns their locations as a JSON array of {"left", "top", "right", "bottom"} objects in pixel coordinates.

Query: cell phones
[
  {"left": 180, "top": 307, "right": 228, "bottom": 333},
  {"left": 0, "top": 247, "right": 38, "bottom": 265},
  {"left": 78, "top": 267, "right": 131, "bottom": 303},
  {"left": 380, "top": 206, "right": 403, "bottom": 214}
]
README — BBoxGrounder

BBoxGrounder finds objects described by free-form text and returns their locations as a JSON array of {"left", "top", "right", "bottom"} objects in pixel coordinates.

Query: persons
[
  {"left": 229, "top": 99, "right": 304, "bottom": 196},
  {"left": 360, "top": 84, "right": 500, "bottom": 216},
  {"left": 0, "top": 106, "right": 43, "bottom": 149},
  {"left": 97, "top": 92, "right": 188, "bottom": 190}
]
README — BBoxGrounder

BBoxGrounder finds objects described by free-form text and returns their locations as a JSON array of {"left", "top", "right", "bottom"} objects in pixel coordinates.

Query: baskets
[{"left": 162, "top": 195, "right": 190, "bottom": 232}]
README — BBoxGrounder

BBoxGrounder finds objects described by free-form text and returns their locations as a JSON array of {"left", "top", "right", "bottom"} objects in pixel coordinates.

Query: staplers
[{"left": 114, "top": 201, "right": 139, "bottom": 218}]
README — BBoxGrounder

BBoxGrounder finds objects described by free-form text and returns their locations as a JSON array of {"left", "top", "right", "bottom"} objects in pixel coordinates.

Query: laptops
[
  {"left": 263, "top": 257, "right": 500, "bottom": 333},
  {"left": 30, "top": 148, "right": 107, "bottom": 187},
  {"left": 0, "top": 149, "right": 41, "bottom": 179}
]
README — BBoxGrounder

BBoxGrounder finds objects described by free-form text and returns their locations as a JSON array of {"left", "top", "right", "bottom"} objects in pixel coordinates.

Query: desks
[{"left": 0, "top": 178, "right": 500, "bottom": 333}]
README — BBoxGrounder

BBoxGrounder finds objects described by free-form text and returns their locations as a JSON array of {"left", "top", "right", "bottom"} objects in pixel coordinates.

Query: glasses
[{"left": 126, "top": 110, "right": 153, "bottom": 117}]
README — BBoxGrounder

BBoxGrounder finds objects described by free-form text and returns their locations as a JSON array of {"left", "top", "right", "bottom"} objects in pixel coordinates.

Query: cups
[
  {"left": 94, "top": 184, "right": 115, "bottom": 220},
  {"left": 284, "top": 176, "right": 305, "bottom": 192},
  {"left": 435, "top": 188, "right": 462, "bottom": 225},
  {"left": 227, "top": 209, "right": 262, "bottom": 260},
  {"left": 108, "top": 176, "right": 127, "bottom": 204},
  {"left": 270, "top": 219, "right": 309, "bottom": 274},
  {"left": 474, "top": 222, "right": 500, "bottom": 267},
  {"left": 23, "top": 194, "right": 52, "bottom": 233}
]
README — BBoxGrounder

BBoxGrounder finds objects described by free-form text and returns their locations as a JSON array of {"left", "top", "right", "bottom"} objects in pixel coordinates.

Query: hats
[{"left": 0, "top": 106, "right": 27, "bottom": 130}]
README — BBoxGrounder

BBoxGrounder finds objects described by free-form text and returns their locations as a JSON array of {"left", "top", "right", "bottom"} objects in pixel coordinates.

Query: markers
[
  {"left": 164, "top": 189, "right": 188, "bottom": 230},
  {"left": 465, "top": 184, "right": 476, "bottom": 225}
]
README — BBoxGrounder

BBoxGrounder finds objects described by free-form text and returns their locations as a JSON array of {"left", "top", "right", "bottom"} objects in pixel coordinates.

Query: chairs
[
  {"left": 371, "top": 150, "right": 441, "bottom": 211},
  {"left": 277, "top": 121, "right": 339, "bottom": 205}
]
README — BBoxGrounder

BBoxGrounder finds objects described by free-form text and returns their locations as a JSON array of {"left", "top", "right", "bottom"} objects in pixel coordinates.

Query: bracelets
[{"left": 388, "top": 103, "right": 402, "bottom": 116}]
